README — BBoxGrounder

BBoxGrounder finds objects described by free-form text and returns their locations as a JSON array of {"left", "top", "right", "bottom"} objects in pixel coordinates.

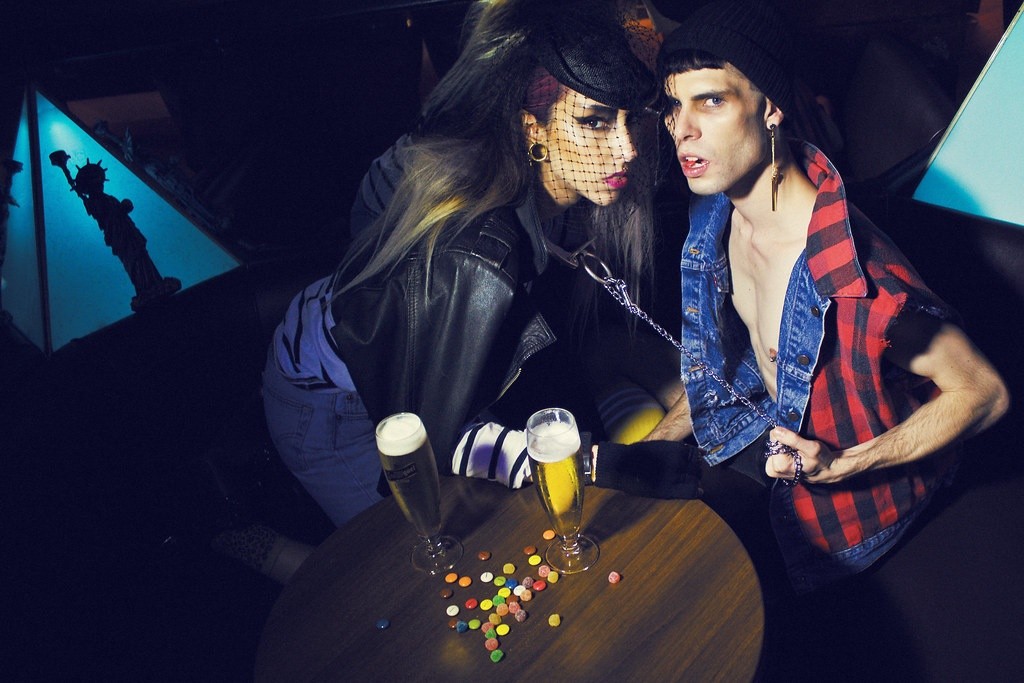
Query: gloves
[{"left": 589, "top": 439, "right": 710, "bottom": 499}]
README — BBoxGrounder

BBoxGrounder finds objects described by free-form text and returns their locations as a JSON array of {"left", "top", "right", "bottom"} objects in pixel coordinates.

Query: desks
[{"left": 253, "top": 470, "right": 764, "bottom": 682}]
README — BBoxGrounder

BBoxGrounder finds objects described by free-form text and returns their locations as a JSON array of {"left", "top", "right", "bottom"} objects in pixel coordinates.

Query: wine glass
[
  {"left": 375, "top": 410, "right": 464, "bottom": 576},
  {"left": 525, "top": 406, "right": 599, "bottom": 573}
]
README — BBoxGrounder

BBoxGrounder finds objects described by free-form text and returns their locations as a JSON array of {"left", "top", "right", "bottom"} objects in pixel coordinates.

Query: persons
[
  {"left": 263, "top": 0, "right": 744, "bottom": 530},
  {"left": 638, "top": 0, "right": 1012, "bottom": 613}
]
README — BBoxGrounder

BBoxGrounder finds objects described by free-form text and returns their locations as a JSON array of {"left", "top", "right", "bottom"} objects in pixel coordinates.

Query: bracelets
[{"left": 579, "top": 432, "right": 593, "bottom": 485}]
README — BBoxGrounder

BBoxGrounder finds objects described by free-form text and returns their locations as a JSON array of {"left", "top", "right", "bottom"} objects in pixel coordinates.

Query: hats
[
  {"left": 532, "top": 0, "right": 664, "bottom": 109},
  {"left": 656, "top": 0, "right": 796, "bottom": 112}
]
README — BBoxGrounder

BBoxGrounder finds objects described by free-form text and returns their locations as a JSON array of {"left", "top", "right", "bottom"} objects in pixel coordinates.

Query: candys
[{"left": 377, "top": 530, "right": 621, "bottom": 664}]
